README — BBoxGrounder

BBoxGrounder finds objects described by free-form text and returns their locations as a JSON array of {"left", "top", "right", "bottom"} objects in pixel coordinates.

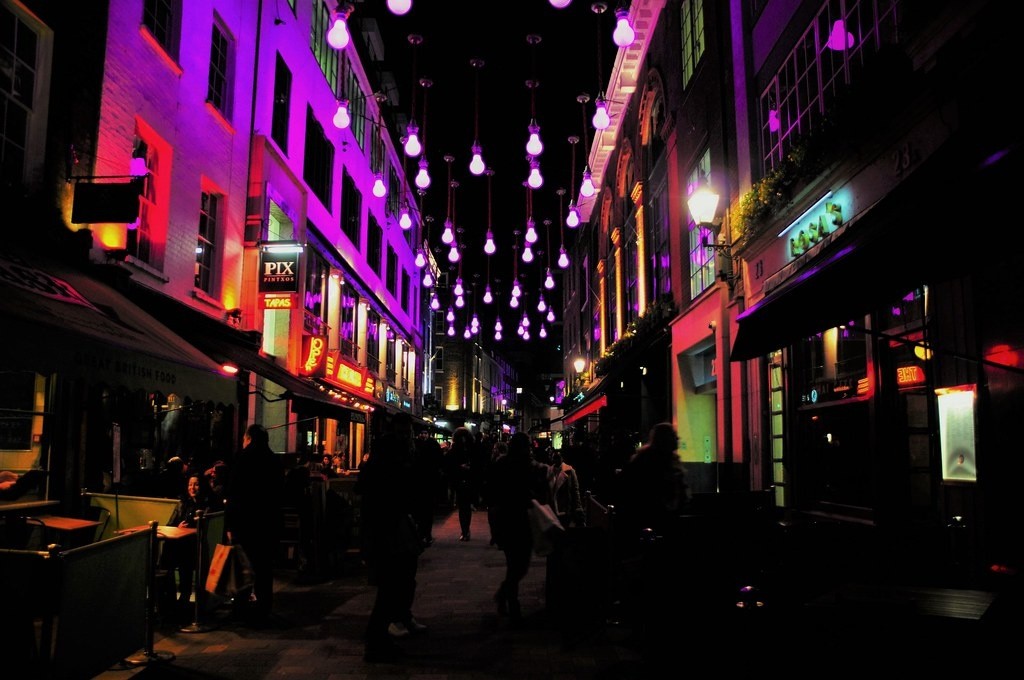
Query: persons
[
  {"left": 450, "top": 421, "right": 695, "bottom": 627},
  {"left": 148, "top": 422, "right": 452, "bottom": 637}
]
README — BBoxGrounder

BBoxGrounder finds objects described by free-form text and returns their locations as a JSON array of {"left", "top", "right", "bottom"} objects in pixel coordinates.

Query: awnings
[
  {"left": 732, "top": 103, "right": 1024, "bottom": 362},
  {"left": 86, "top": 249, "right": 263, "bottom": 352},
  {"left": 0, "top": 257, "right": 242, "bottom": 382},
  {"left": 204, "top": 337, "right": 450, "bottom": 435}
]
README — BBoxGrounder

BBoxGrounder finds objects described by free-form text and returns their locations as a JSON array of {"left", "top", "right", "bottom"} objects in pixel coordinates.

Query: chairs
[
  {"left": 0, "top": 517, "right": 45, "bottom": 552},
  {"left": 72, "top": 506, "right": 112, "bottom": 543}
]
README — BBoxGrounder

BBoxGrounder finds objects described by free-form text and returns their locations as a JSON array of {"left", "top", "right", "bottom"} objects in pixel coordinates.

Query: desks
[
  {"left": 27, "top": 512, "right": 104, "bottom": 549},
  {"left": 114, "top": 522, "right": 197, "bottom": 561}
]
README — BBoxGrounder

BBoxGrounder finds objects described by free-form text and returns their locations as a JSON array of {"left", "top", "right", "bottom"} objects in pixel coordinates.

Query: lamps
[
  {"left": 254, "top": 237, "right": 307, "bottom": 257},
  {"left": 685, "top": 169, "right": 732, "bottom": 261},
  {"left": 572, "top": 357, "right": 592, "bottom": 383}
]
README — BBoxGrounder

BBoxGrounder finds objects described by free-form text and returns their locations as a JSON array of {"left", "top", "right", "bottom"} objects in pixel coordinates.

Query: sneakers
[{"left": 388, "top": 619, "right": 426, "bottom": 637}]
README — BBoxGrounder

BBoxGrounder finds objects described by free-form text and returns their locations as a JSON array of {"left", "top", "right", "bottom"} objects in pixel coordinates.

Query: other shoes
[
  {"left": 494, "top": 582, "right": 522, "bottom": 620},
  {"left": 361, "top": 644, "right": 407, "bottom": 662},
  {"left": 460, "top": 532, "right": 471, "bottom": 541}
]
